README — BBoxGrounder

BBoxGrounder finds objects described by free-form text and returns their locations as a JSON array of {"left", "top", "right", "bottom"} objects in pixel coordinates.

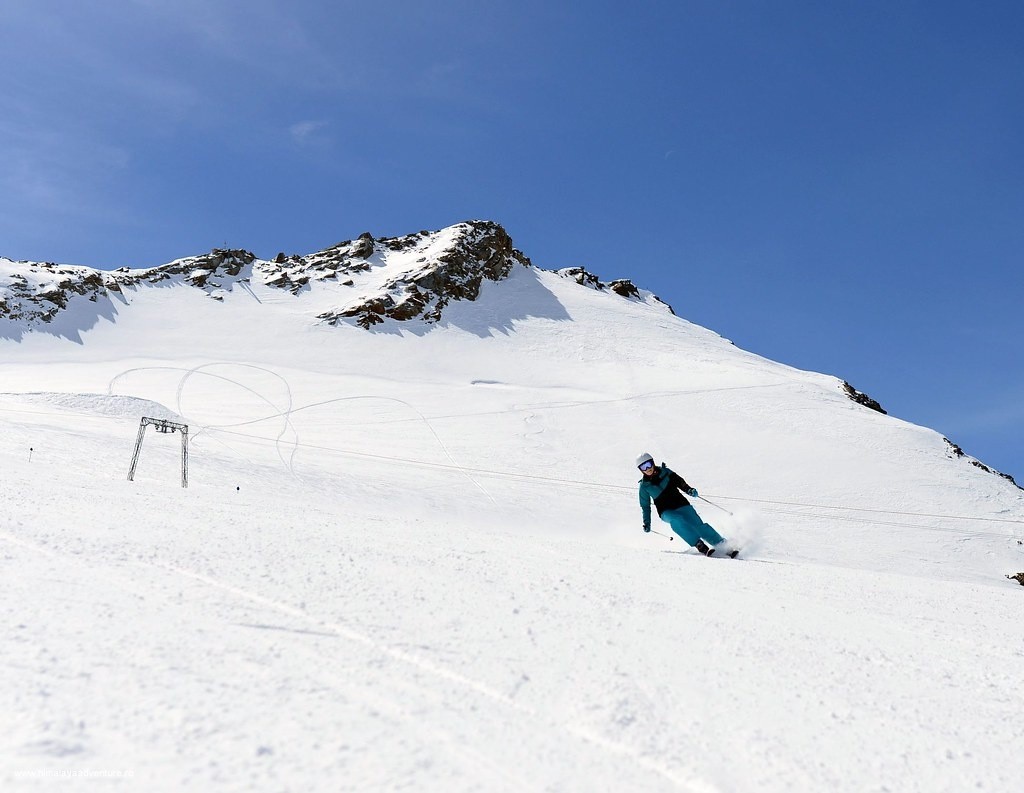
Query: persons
[{"left": 636, "top": 453, "right": 739, "bottom": 559}]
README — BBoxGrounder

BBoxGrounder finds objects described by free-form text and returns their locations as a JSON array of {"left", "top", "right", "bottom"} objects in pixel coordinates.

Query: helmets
[{"left": 635, "top": 452, "right": 653, "bottom": 468}]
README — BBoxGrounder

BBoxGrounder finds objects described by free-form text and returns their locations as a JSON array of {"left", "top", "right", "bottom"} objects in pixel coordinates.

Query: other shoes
[{"left": 695, "top": 539, "right": 709, "bottom": 555}]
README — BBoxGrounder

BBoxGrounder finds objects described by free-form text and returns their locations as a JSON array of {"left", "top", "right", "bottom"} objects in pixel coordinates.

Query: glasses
[{"left": 639, "top": 459, "right": 654, "bottom": 471}]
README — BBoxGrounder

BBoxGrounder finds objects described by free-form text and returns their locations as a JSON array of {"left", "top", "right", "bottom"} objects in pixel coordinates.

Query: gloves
[
  {"left": 687, "top": 487, "right": 698, "bottom": 497},
  {"left": 643, "top": 524, "right": 650, "bottom": 533}
]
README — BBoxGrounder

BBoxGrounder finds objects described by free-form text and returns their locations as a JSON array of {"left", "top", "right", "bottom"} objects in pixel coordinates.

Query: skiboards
[{"left": 705, "top": 548, "right": 739, "bottom": 559}]
